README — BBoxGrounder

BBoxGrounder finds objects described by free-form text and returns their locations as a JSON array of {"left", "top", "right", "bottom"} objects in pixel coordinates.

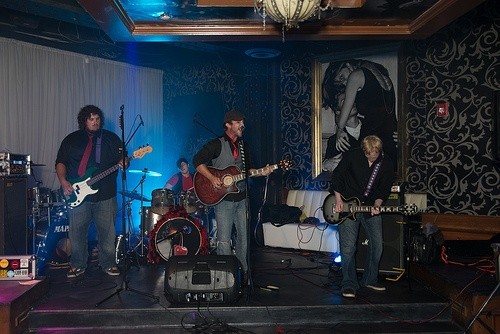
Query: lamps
[{"left": 253, "top": 0, "right": 334, "bottom": 43}]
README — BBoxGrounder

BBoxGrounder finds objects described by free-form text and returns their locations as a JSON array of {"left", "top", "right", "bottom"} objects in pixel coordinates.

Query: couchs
[{"left": 261, "top": 189, "right": 428, "bottom": 271}]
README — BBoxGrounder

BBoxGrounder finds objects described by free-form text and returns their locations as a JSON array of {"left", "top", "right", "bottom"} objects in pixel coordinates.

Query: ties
[{"left": 77, "top": 133, "right": 95, "bottom": 177}]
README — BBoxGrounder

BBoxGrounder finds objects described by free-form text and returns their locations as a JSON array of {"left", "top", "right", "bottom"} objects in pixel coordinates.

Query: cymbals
[
  {"left": 128, "top": 170, "right": 162, "bottom": 176},
  {"left": 118, "top": 191, "right": 152, "bottom": 203}
]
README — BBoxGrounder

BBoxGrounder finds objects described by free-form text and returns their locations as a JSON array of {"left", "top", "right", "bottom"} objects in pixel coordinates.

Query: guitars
[
  {"left": 193, "top": 158, "right": 294, "bottom": 207},
  {"left": 322, "top": 193, "right": 419, "bottom": 226},
  {"left": 59, "top": 143, "right": 155, "bottom": 208}
]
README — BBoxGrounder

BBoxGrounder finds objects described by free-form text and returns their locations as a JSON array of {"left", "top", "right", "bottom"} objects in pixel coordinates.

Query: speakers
[
  {"left": 164, "top": 254, "right": 244, "bottom": 307},
  {"left": 0, "top": 173, "right": 35, "bottom": 255},
  {"left": 355, "top": 213, "right": 421, "bottom": 274}
]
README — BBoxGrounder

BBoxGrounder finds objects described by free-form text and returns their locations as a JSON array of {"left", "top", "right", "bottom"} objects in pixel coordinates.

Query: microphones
[
  {"left": 239, "top": 125, "right": 245, "bottom": 130},
  {"left": 139, "top": 114, "right": 144, "bottom": 126},
  {"left": 193, "top": 113, "right": 197, "bottom": 126}
]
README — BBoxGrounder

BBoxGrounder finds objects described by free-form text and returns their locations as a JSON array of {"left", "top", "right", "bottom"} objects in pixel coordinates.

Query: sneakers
[
  {"left": 104, "top": 266, "right": 121, "bottom": 276},
  {"left": 67, "top": 266, "right": 85, "bottom": 278}
]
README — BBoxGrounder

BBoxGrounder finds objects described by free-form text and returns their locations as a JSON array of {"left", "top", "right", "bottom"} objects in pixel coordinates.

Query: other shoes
[
  {"left": 342, "top": 288, "right": 356, "bottom": 298},
  {"left": 365, "top": 282, "right": 387, "bottom": 291}
]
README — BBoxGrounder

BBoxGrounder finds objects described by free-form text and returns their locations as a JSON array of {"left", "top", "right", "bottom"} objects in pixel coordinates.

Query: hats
[{"left": 223, "top": 110, "right": 247, "bottom": 123}]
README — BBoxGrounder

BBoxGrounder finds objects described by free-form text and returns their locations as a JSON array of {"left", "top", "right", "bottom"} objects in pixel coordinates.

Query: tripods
[{"left": 95, "top": 104, "right": 160, "bottom": 307}]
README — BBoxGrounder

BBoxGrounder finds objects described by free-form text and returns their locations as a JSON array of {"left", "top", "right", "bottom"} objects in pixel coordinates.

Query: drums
[
  {"left": 148, "top": 188, "right": 176, "bottom": 215},
  {"left": 176, "top": 190, "right": 200, "bottom": 214},
  {"left": 27, "top": 186, "right": 51, "bottom": 209},
  {"left": 51, "top": 188, "right": 66, "bottom": 208},
  {"left": 153, "top": 215, "right": 202, "bottom": 262},
  {"left": 136, "top": 205, "right": 164, "bottom": 239},
  {"left": 34, "top": 214, "right": 74, "bottom": 266}
]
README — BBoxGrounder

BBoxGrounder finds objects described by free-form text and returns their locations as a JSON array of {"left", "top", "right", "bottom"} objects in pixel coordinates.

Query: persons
[
  {"left": 191, "top": 110, "right": 273, "bottom": 285},
  {"left": 332, "top": 135, "right": 394, "bottom": 297},
  {"left": 164, "top": 156, "right": 194, "bottom": 192},
  {"left": 55, "top": 105, "right": 129, "bottom": 277},
  {"left": 321, "top": 58, "right": 398, "bottom": 173}
]
